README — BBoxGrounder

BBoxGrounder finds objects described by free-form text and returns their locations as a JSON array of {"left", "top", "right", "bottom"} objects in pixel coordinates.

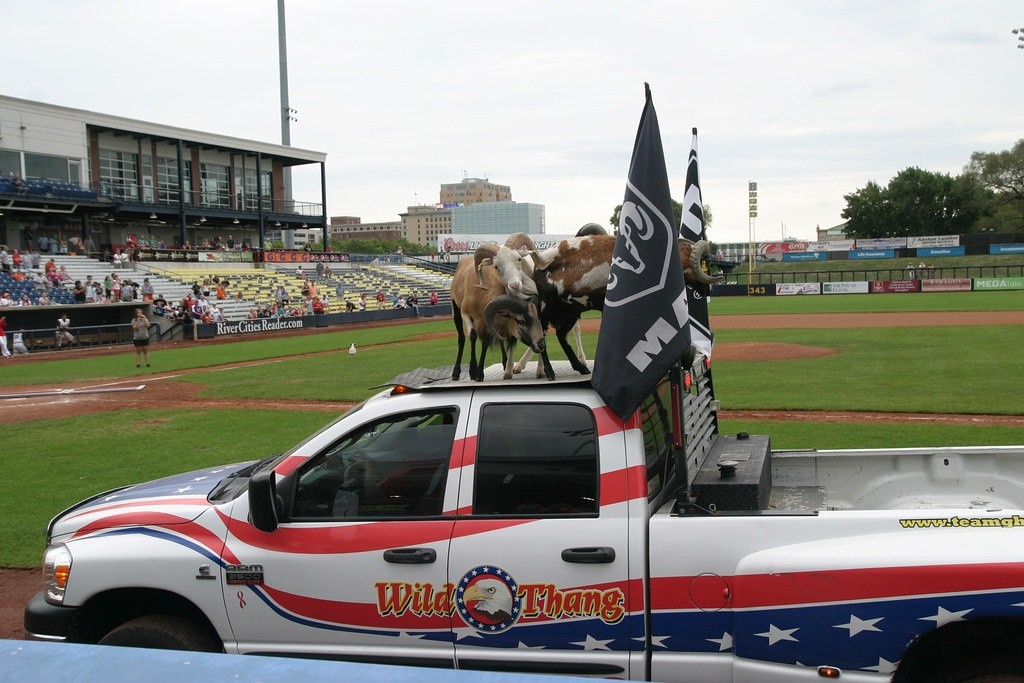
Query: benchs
[{"left": 39, "top": 252, "right": 456, "bottom": 326}]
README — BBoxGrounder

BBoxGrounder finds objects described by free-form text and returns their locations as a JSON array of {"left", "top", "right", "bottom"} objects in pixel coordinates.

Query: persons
[
  {"left": 56, "top": 313, "right": 74, "bottom": 348},
  {"left": 12, "top": 329, "right": 28, "bottom": 355},
  {"left": 0, "top": 171, "right": 441, "bottom": 319},
  {"left": 0, "top": 317, "right": 11, "bottom": 356},
  {"left": 131, "top": 309, "right": 151, "bottom": 368}
]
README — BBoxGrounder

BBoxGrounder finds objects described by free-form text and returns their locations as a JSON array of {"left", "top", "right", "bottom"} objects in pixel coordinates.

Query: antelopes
[{"left": 450, "top": 222, "right": 724, "bottom": 383}]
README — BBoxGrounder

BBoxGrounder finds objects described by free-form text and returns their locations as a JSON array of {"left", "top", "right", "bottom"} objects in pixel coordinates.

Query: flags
[
  {"left": 590, "top": 87, "right": 692, "bottom": 424},
  {"left": 677, "top": 135, "right": 713, "bottom": 360}
]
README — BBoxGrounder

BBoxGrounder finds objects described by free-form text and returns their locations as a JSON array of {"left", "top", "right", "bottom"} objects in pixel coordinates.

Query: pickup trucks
[{"left": 21, "top": 356, "right": 1023, "bottom": 683}]
